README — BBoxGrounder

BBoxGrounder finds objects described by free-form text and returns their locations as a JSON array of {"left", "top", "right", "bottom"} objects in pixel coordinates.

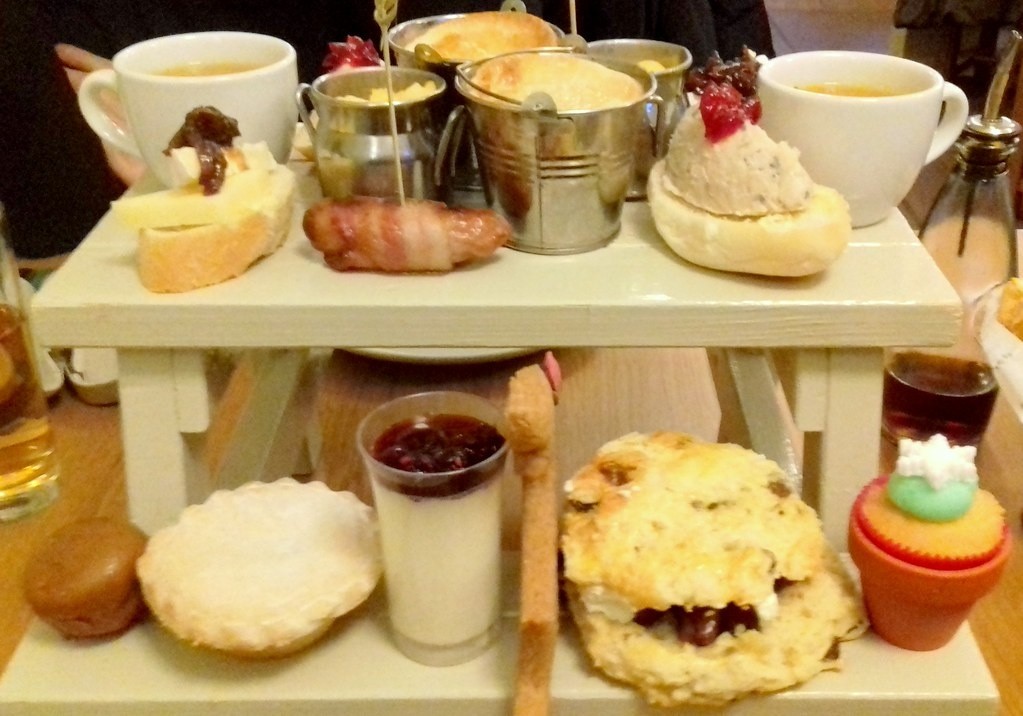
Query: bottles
[{"left": 878, "top": 30, "right": 1022, "bottom": 450}]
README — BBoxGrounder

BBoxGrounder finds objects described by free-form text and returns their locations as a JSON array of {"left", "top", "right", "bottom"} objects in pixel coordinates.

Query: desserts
[
  {"left": 852, "top": 434, "right": 1013, "bottom": 569},
  {"left": 466, "top": 50, "right": 645, "bottom": 113},
  {"left": 134, "top": 475, "right": 384, "bottom": 661},
  {"left": 403, "top": 8, "right": 557, "bottom": 64},
  {"left": 111, "top": 108, "right": 297, "bottom": 293},
  {"left": 645, "top": 89, "right": 852, "bottom": 277},
  {"left": 561, "top": 427, "right": 868, "bottom": 703}
]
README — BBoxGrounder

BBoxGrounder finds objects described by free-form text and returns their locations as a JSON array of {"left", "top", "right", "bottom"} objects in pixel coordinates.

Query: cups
[
  {"left": 579, "top": 38, "right": 693, "bottom": 198},
  {"left": 758, "top": 51, "right": 968, "bottom": 229},
  {"left": 79, "top": 31, "right": 299, "bottom": 185},
  {"left": 295, "top": 66, "right": 447, "bottom": 200},
  {"left": 356, "top": 390, "right": 513, "bottom": 666}
]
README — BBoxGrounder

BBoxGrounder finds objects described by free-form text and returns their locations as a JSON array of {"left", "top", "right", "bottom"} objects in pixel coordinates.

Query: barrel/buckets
[
  {"left": 388, "top": 12, "right": 568, "bottom": 191},
  {"left": 454, "top": 51, "right": 656, "bottom": 255}
]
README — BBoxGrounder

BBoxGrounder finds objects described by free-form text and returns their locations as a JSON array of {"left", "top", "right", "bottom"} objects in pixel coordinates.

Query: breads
[{"left": 22, "top": 516, "right": 151, "bottom": 640}]
